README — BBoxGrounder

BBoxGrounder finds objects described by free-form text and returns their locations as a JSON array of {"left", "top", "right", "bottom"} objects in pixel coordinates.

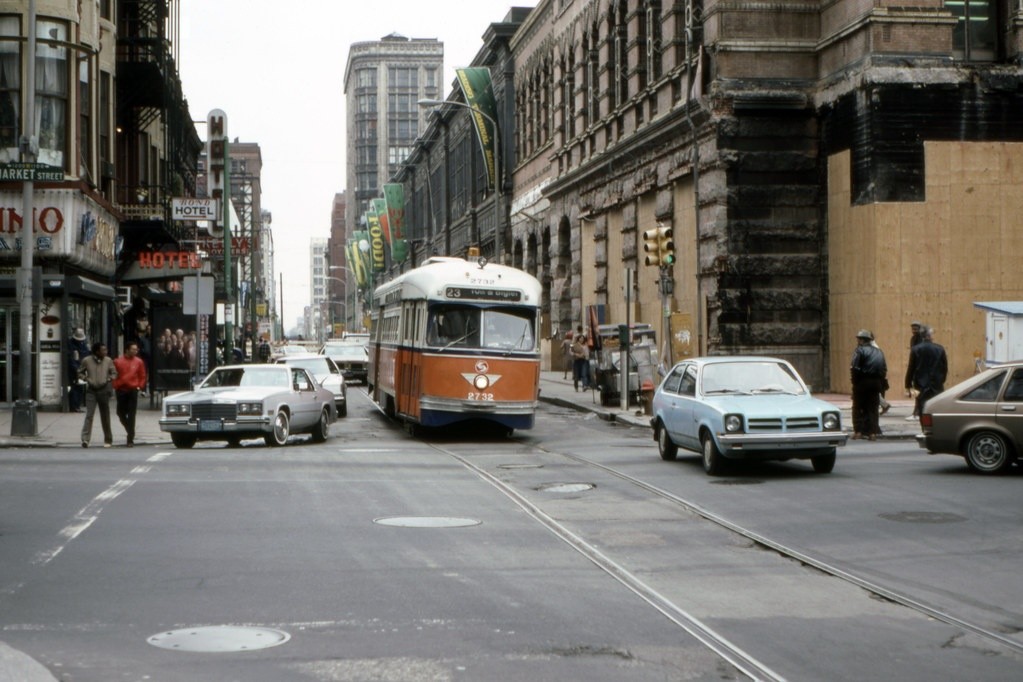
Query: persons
[
  {"left": 77, "top": 342, "right": 146, "bottom": 447},
  {"left": 257, "top": 335, "right": 271, "bottom": 362},
  {"left": 216, "top": 336, "right": 252, "bottom": 365},
  {"left": 141, "top": 325, "right": 151, "bottom": 396},
  {"left": 905, "top": 322, "right": 949, "bottom": 438},
  {"left": 69, "top": 328, "right": 88, "bottom": 412},
  {"left": 850, "top": 330, "right": 891, "bottom": 440},
  {"left": 561, "top": 327, "right": 593, "bottom": 391},
  {"left": 298, "top": 335, "right": 304, "bottom": 346},
  {"left": 158, "top": 328, "right": 196, "bottom": 369}
]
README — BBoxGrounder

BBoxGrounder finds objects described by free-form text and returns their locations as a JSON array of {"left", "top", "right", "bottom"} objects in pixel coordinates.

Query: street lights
[
  {"left": 417, "top": 97, "right": 500, "bottom": 264},
  {"left": 325, "top": 266, "right": 358, "bottom": 333}
]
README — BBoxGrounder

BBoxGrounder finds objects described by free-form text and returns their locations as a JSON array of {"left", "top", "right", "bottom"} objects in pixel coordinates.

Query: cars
[
  {"left": 158, "top": 364, "right": 337, "bottom": 449},
  {"left": 268, "top": 333, "right": 370, "bottom": 417},
  {"left": 915, "top": 359, "right": 1023, "bottom": 476},
  {"left": 650, "top": 355, "right": 851, "bottom": 477}
]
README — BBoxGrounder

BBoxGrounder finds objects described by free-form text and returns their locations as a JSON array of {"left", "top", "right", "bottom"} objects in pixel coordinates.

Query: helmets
[{"left": 857, "top": 330, "right": 872, "bottom": 339}]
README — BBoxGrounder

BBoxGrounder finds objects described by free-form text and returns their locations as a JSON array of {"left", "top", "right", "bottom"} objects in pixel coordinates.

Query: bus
[{"left": 367, "top": 248, "right": 544, "bottom": 438}]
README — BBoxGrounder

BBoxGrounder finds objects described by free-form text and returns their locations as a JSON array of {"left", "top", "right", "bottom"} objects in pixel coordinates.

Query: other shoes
[
  {"left": 575, "top": 387, "right": 579, "bottom": 392},
  {"left": 905, "top": 415, "right": 919, "bottom": 421},
  {"left": 71, "top": 407, "right": 83, "bottom": 412},
  {"left": 852, "top": 432, "right": 862, "bottom": 439},
  {"left": 82, "top": 441, "right": 89, "bottom": 448},
  {"left": 104, "top": 443, "right": 111, "bottom": 447},
  {"left": 128, "top": 440, "right": 133, "bottom": 447},
  {"left": 583, "top": 387, "right": 588, "bottom": 392},
  {"left": 868, "top": 434, "right": 876, "bottom": 440},
  {"left": 880, "top": 404, "right": 891, "bottom": 416}
]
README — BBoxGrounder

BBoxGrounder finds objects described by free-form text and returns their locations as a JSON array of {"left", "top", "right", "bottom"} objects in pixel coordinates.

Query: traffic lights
[
  {"left": 658, "top": 226, "right": 676, "bottom": 268},
  {"left": 643, "top": 228, "right": 660, "bottom": 267}
]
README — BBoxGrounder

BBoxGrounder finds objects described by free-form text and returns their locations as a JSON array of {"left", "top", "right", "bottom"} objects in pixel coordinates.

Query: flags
[
  {"left": 347, "top": 184, "right": 408, "bottom": 288},
  {"left": 456, "top": 68, "right": 503, "bottom": 190}
]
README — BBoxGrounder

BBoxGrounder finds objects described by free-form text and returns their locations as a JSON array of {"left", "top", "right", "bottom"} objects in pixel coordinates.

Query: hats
[
  {"left": 911, "top": 322, "right": 921, "bottom": 327},
  {"left": 919, "top": 326, "right": 932, "bottom": 338},
  {"left": 72, "top": 328, "right": 86, "bottom": 341}
]
